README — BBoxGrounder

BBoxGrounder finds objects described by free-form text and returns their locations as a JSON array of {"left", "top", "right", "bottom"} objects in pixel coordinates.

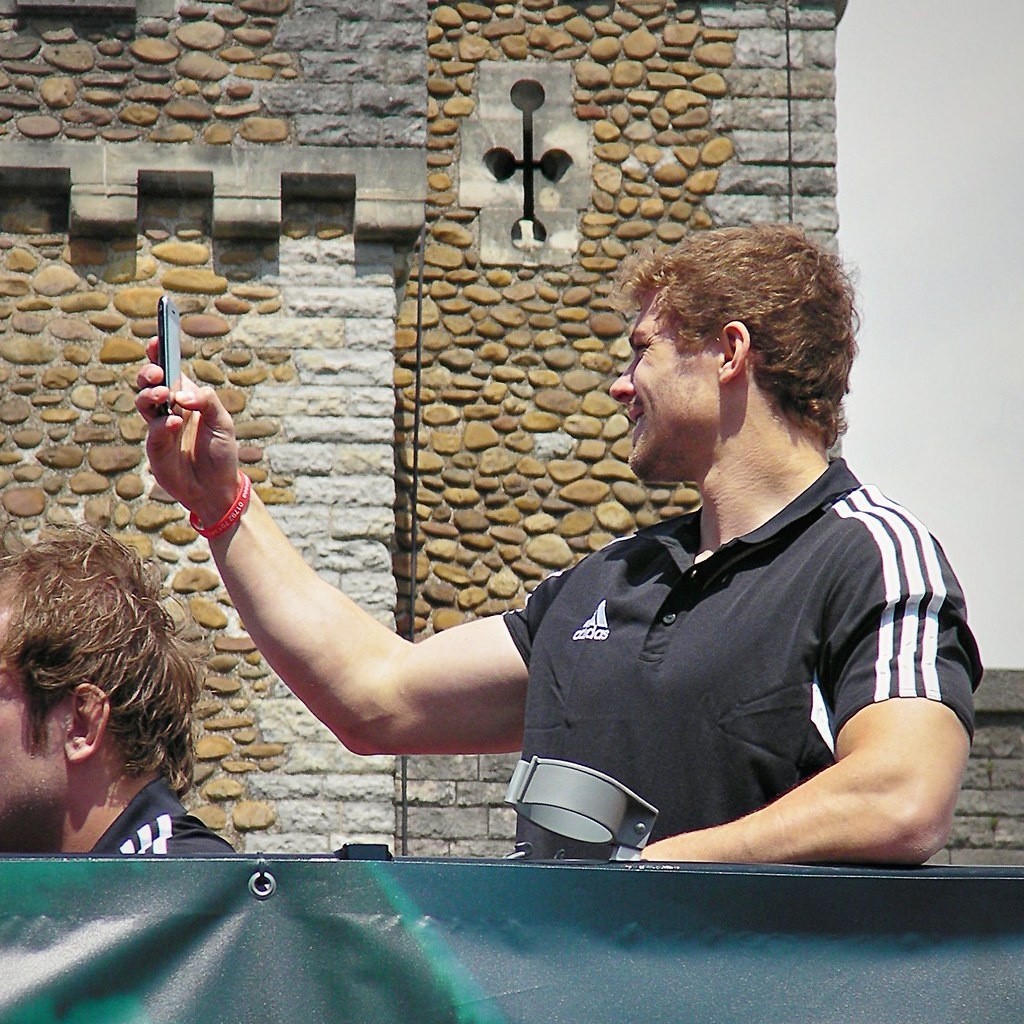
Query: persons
[
  {"left": 0, "top": 528, "right": 236, "bottom": 854},
  {"left": 135, "top": 223, "right": 982, "bottom": 866}
]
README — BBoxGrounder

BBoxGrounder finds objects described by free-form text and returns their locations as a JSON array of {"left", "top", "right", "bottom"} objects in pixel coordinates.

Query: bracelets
[{"left": 189, "top": 472, "right": 251, "bottom": 538}]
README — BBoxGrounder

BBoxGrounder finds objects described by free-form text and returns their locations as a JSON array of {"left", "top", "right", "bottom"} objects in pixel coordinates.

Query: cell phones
[{"left": 157, "top": 295, "right": 182, "bottom": 420}]
